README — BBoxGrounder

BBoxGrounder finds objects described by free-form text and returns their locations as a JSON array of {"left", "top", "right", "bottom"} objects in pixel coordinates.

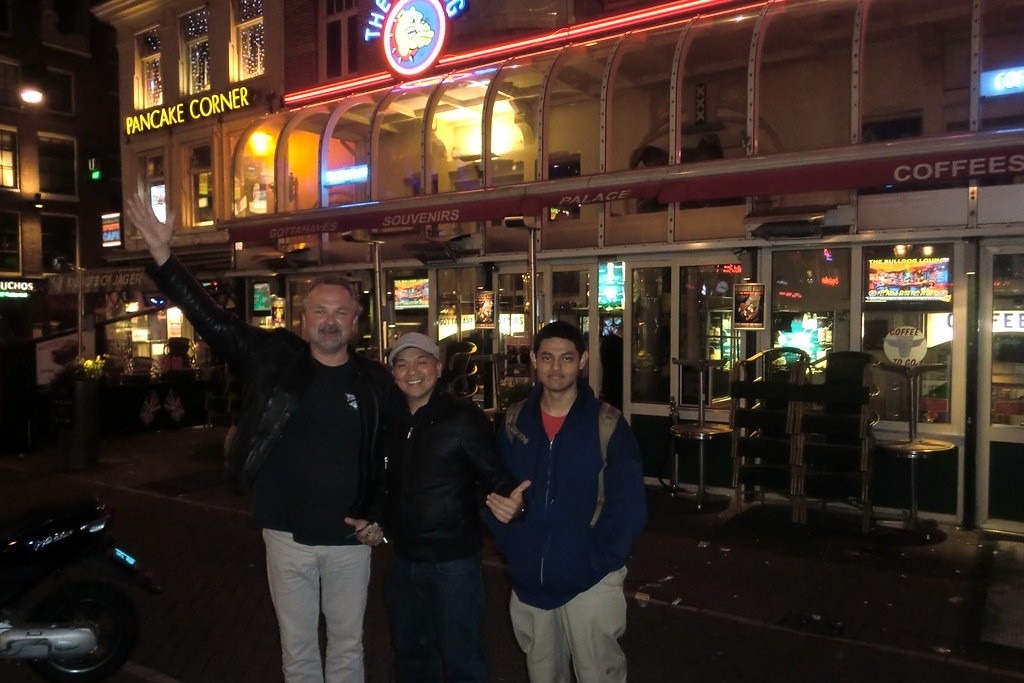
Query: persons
[
  {"left": 600, "top": 321, "right": 624, "bottom": 413},
  {"left": 124, "top": 192, "right": 395, "bottom": 683},
  {"left": 483, "top": 321, "right": 649, "bottom": 683},
  {"left": 343, "top": 333, "right": 533, "bottom": 683}
]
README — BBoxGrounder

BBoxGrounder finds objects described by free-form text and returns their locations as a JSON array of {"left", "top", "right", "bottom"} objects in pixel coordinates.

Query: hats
[{"left": 387, "top": 332, "right": 440, "bottom": 367}]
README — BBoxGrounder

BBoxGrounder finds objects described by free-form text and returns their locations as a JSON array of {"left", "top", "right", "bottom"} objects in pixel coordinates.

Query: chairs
[
  {"left": 206, "top": 366, "right": 237, "bottom": 430},
  {"left": 445, "top": 339, "right": 480, "bottom": 403},
  {"left": 727, "top": 346, "right": 883, "bottom": 535}
]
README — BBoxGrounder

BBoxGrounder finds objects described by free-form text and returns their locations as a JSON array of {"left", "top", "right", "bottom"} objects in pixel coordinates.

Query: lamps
[
  {"left": 253, "top": 250, "right": 298, "bottom": 272},
  {"left": 15, "top": 62, "right": 49, "bottom": 110},
  {"left": 749, "top": 221, "right": 823, "bottom": 244},
  {"left": 401, "top": 239, "right": 458, "bottom": 265},
  {"left": 32, "top": 193, "right": 44, "bottom": 208}
]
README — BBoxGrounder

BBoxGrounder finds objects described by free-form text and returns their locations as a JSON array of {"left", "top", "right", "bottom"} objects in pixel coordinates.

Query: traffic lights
[{"left": 90, "top": 170, "right": 102, "bottom": 181}]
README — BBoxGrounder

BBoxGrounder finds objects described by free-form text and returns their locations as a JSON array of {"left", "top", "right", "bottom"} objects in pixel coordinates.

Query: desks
[
  {"left": 871, "top": 358, "right": 961, "bottom": 456},
  {"left": 873, "top": 454, "right": 962, "bottom": 538},
  {"left": 670, "top": 356, "right": 733, "bottom": 436},
  {"left": 669, "top": 433, "right": 733, "bottom": 513}
]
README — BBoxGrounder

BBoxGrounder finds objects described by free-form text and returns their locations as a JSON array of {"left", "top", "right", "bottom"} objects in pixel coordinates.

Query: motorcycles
[{"left": 0, "top": 490, "right": 162, "bottom": 683}]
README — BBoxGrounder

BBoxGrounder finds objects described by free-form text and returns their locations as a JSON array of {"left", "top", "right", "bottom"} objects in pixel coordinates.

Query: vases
[{"left": 130, "top": 356, "right": 153, "bottom": 376}]
